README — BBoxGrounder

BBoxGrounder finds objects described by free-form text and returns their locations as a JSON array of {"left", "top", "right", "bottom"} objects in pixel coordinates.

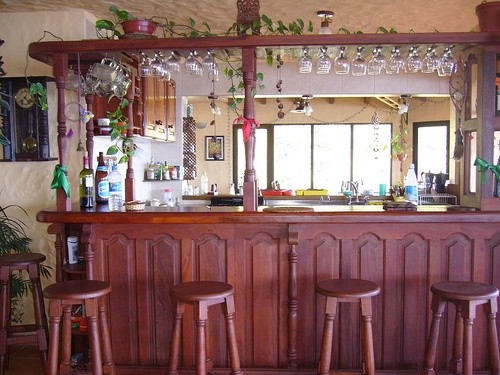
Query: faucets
[{"left": 346, "top": 180, "right": 359, "bottom": 202}]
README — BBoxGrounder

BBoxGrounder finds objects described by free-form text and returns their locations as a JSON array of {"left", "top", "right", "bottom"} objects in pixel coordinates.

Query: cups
[
  {"left": 379, "top": 183, "right": 387, "bottom": 195},
  {"left": 65, "top": 57, "right": 132, "bottom": 100}
]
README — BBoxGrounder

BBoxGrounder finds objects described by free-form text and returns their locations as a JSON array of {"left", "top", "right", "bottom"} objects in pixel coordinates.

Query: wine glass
[
  {"left": 138, "top": 50, "right": 219, "bottom": 82},
  {"left": 352, "top": 46, "right": 367, "bottom": 76},
  {"left": 368, "top": 44, "right": 458, "bottom": 77},
  {"left": 298, "top": 46, "right": 313, "bottom": 74},
  {"left": 315, "top": 46, "right": 332, "bottom": 75},
  {"left": 334, "top": 46, "right": 350, "bottom": 75}
]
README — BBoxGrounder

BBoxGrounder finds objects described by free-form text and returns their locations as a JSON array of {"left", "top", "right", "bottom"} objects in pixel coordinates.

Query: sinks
[{"left": 318, "top": 201, "right": 365, "bottom": 205}]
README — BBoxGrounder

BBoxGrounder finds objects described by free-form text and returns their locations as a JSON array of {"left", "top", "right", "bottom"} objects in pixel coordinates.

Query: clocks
[{"left": 0, "top": 76, "right": 58, "bottom": 162}]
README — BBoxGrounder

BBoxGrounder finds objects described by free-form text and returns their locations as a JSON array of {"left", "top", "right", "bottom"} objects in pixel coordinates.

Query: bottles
[
  {"left": 201, "top": 172, "right": 208, "bottom": 195},
  {"left": 147, "top": 160, "right": 180, "bottom": 180},
  {"left": 109, "top": 166, "right": 123, "bottom": 212},
  {"left": 184, "top": 179, "right": 199, "bottom": 195},
  {"left": 164, "top": 188, "right": 179, "bottom": 207},
  {"left": 239, "top": 177, "right": 243, "bottom": 195},
  {"left": 79, "top": 157, "right": 94, "bottom": 208},
  {"left": 67, "top": 236, "right": 79, "bottom": 265},
  {"left": 229, "top": 182, "right": 235, "bottom": 195},
  {"left": 404, "top": 164, "right": 418, "bottom": 206},
  {"left": 95, "top": 151, "right": 109, "bottom": 204}
]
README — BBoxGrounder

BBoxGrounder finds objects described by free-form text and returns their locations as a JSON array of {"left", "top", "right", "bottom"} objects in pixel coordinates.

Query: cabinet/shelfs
[
  {"left": 36, "top": 206, "right": 500, "bottom": 375},
  {"left": 92, "top": 76, "right": 176, "bottom": 142}
]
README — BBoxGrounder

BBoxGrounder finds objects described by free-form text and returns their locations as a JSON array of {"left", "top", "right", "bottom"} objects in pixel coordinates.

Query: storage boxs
[{"left": 261, "top": 189, "right": 328, "bottom": 196}]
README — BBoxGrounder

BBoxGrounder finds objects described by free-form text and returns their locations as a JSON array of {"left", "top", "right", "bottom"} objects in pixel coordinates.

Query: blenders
[
  {"left": 421, "top": 169, "right": 435, "bottom": 189},
  {"left": 433, "top": 171, "right": 449, "bottom": 194}
]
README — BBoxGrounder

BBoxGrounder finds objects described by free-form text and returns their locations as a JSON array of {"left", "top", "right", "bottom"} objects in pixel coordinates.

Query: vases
[{"left": 118, "top": 19, "right": 158, "bottom": 39}]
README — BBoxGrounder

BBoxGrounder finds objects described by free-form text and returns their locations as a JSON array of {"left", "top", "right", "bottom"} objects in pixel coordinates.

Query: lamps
[
  {"left": 292, "top": 95, "right": 314, "bottom": 117},
  {"left": 317, "top": 11, "right": 334, "bottom": 34},
  {"left": 398, "top": 95, "right": 412, "bottom": 115}
]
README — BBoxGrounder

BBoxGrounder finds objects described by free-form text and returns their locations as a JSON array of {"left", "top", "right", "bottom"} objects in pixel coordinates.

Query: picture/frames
[{"left": 205, "top": 136, "right": 224, "bottom": 161}]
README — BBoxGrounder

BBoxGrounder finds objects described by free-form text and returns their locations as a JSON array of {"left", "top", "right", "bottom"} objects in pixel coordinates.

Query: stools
[
  {"left": 42, "top": 280, "right": 115, "bottom": 375},
  {"left": 317, "top": 278, "right": 380, "bottom": 375},
  {"left": 168, "top": 281, "right": 243, "bottom": 375},
  {"left": 0, "top": 253, "right": 50, "bottom": 375},
  {"left": 422, "top": 281, "right": 500, "bottom": 375}
]
